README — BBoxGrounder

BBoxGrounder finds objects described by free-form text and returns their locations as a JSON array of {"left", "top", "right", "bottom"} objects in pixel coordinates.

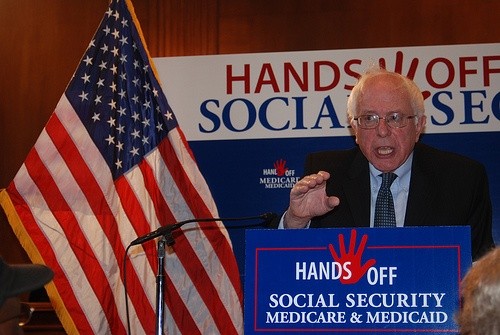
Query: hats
[{"left": 0, "top": 257, "right": 55, "bottom": 300}]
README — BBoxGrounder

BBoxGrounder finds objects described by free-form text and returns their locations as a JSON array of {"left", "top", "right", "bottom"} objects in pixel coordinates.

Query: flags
[{"left": 0, "top": 0, "right": 245, "bottom": 335}]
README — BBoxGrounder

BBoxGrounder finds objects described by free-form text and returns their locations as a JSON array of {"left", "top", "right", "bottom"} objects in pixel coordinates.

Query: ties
[{"left": 374, "top": 173, "right": 398, "bottom": 227}]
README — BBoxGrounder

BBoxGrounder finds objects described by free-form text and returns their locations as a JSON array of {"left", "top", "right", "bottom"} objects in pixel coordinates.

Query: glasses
[
  {"left": 354, "top": 113, "right": 417, "bottom": 130},
  {"left": 0, "top": 303, "right": 34, "bottom": 327}
]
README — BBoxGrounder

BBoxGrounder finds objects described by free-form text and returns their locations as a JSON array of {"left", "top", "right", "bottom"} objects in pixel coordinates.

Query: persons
[
  {"left": 263, "top": 67, "right": 496, "bottom": 261},
  {"left": 453, "top": 247, "right": 500, "bottom": 335}
]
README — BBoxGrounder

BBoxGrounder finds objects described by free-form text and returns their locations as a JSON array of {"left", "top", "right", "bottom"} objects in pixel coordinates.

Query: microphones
[{"left": 130, "top": 210, "right": 274, "bottom": 246}]
